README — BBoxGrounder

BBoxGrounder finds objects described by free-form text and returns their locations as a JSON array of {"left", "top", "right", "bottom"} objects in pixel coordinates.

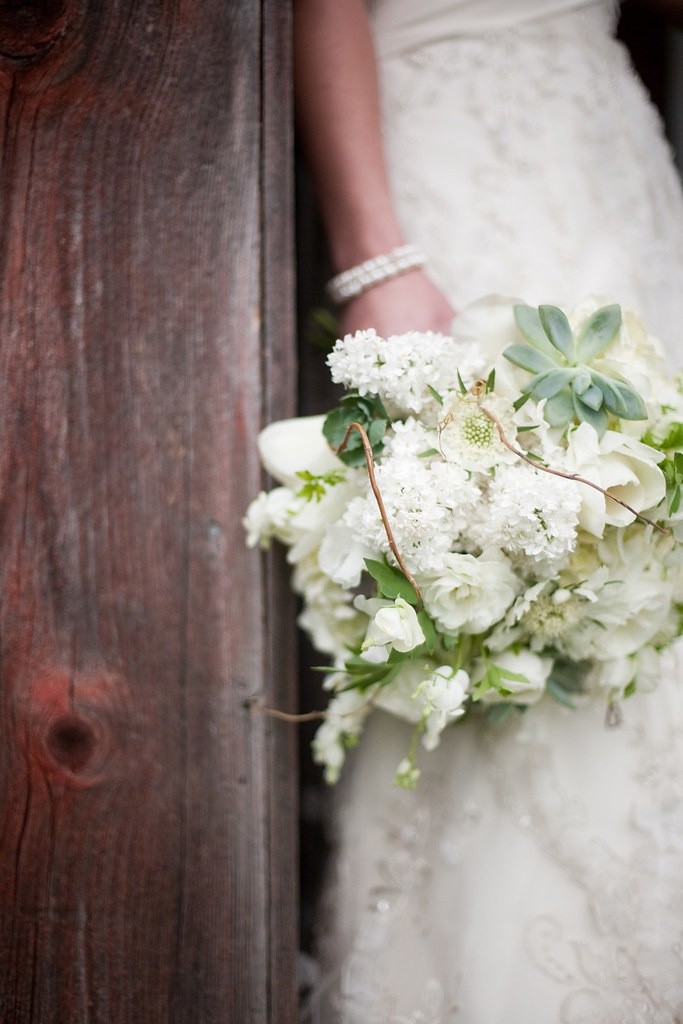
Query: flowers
[{"left": 240, "top": 300, "right": 683, "bottom": 788}]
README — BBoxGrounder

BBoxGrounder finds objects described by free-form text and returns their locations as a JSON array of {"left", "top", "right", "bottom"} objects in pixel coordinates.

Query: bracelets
[{"left": 326, "top": 242, "right": 425, "bottom": 301}]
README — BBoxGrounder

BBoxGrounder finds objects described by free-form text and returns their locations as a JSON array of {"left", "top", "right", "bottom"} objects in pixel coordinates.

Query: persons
[{"left": 293, "top": 0, "right": 683, "bottom": 1024}]
